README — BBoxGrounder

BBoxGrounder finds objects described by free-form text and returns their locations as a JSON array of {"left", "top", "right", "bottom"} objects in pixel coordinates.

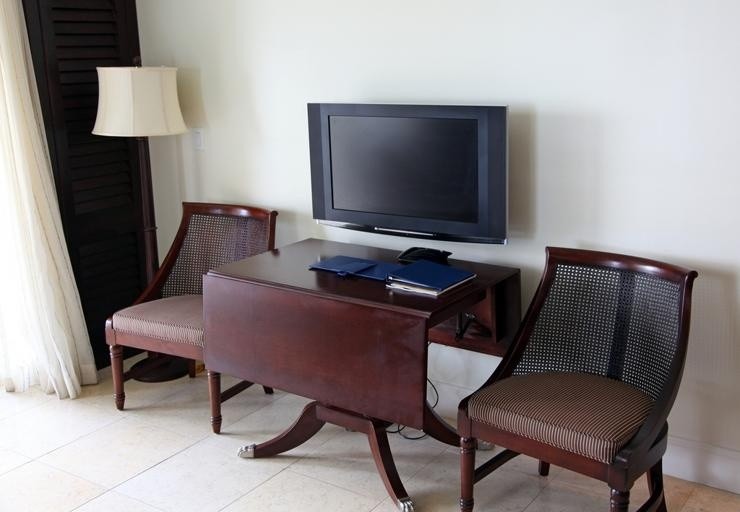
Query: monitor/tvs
[{"left": 306, "top": 102, "right": 510, "bottom": 245}]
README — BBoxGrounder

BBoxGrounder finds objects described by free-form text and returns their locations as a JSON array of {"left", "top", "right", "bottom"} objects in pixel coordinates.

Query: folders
[{"left": 384, "top": 259, "right": 478, "bottom": 296}]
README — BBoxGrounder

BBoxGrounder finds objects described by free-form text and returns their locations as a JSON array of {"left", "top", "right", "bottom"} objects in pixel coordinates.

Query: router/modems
[{"left": 397, "top": 247, "right": 452, "bottom": 265}]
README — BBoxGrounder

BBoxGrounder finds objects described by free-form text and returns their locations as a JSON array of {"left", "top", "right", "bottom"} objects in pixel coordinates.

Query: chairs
[
  {"left": 457, "top": 246, "right": 698, "bottom": 512},
  {"left": 105, "top": 201, "right": 278, "bottom": 434}
]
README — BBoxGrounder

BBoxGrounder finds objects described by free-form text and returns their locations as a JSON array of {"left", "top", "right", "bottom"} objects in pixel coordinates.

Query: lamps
[{"left": 90, "top": 67, "right": 191, "bottom": 382}]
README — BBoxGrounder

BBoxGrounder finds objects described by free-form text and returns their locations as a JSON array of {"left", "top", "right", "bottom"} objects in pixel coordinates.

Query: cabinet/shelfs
[{"left": 202, "top": 237, "right": 521, "bottom": 512}]
